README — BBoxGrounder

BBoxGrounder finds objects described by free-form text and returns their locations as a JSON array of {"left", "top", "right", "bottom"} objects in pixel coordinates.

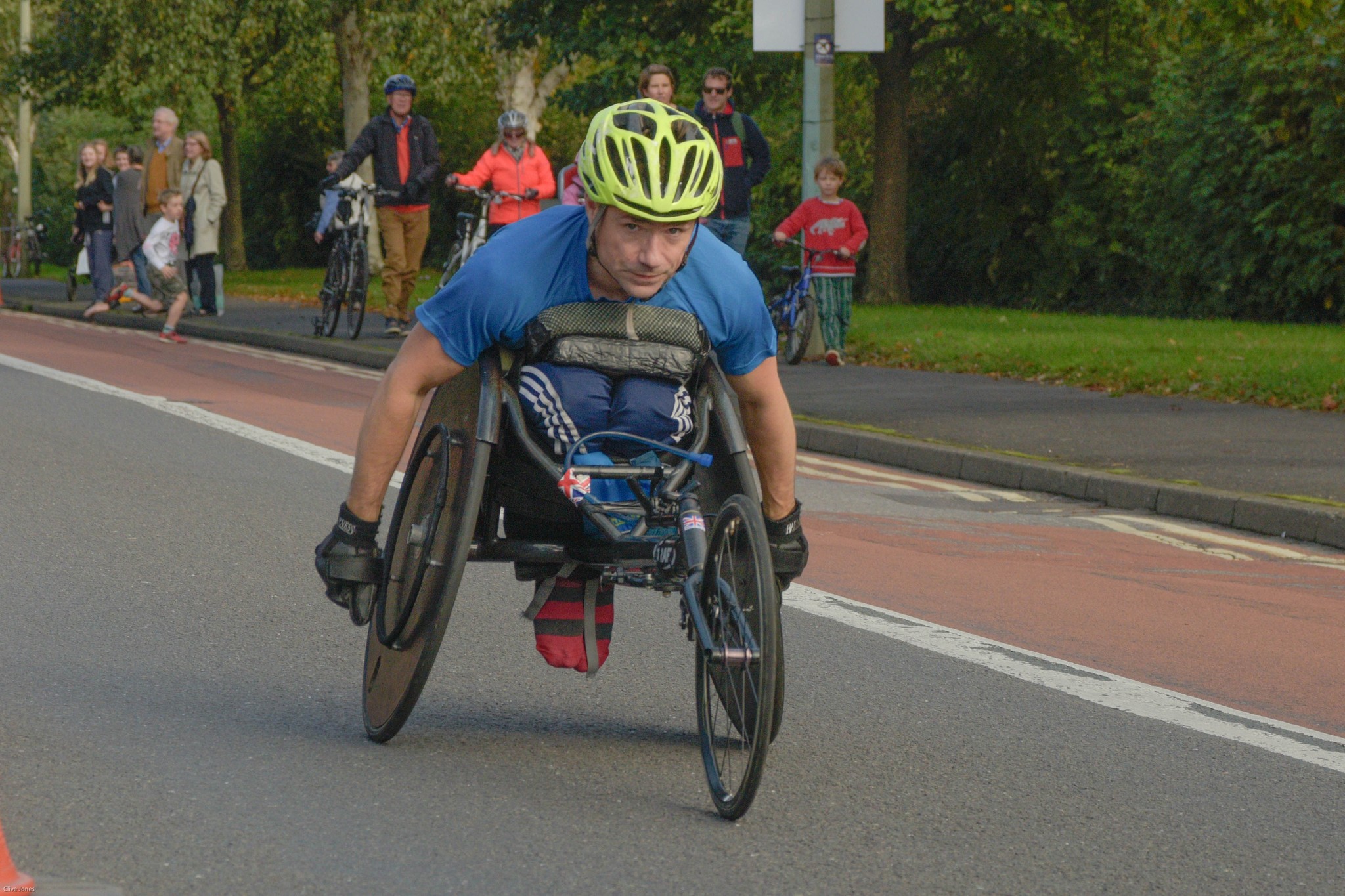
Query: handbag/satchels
[
  {"left": 184, "top": 197, "right": 196, "bottom": 246},
  {"left": 76, "top": 247, "right": 90, "bottom": 275}
]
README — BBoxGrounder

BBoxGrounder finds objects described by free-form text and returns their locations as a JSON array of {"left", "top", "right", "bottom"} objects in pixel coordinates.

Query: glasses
[
  {"left": 505, "top": 132, "right": 523, "bottom": 138},
  {"left": 703, "top": 86, "right": 724, "bottom": 94}
]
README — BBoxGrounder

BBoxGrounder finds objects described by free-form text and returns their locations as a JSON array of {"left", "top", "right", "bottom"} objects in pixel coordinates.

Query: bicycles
[
  {"left": 436, "top": 185, "right": 535, "bottom": 295},
  {"left": 0, "top": 210, "right": 51, "bottom": 279},
  {"left": 764, "top": 236, "right": 858, "bottom": 366},
  {"left": 317, "top": 182, "right": 399, "bottom": 341}
]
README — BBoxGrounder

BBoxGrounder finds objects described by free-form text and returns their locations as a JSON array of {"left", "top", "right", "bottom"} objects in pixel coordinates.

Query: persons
[
  {"left": 637, "top": 64, "right": 692, "bottom": 115},
  {"left": 67, "top": 107, "right": 182, "bottom": 319},
  {"left": 313, "top": 146, "right": 374, "bottom": 311},
  {"left": 26, "top": 216, "right": 47, "bottom": 277},
  {"left": 315, "top": 99, "right": 811, "bottom": 672},
  {"left": 317, "top": 75, "right": 440, "bottom": 340},
  {"left": 445, "top": 109, "right": 556, "bottom": 247},
  {"left": 110, "top": 191, "right": 189, "bottom": 345},
  {"left": 175, "top": 130, "right": 228, "bottom": 315},
  {"left": 773, "top": 156, "right": 868, "bottom": 366},
  {"left": 685, "top": 67, "right": 771, "bottom": 256}
]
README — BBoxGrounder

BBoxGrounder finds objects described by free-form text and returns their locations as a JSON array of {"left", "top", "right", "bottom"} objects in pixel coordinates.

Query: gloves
[
  {"left": 315, "top": 503, "right": 383, "bottom": 624},
  {"left": 525, "top": 187, "right": 538, "bottom": 199},
  {"left": 763, "top": 499, "right": 808, "bottom": 590},
  {"left": 446, "top": 174, "right": 458, "bottom": 191},
  {"left": 401, "top": 175, "right": 424, "bottom": 201},
  {"left": 318, "top": 174, "right": 340, "bottom": 194}
]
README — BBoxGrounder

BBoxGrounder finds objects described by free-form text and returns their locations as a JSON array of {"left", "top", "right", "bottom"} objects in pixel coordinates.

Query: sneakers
[
  {"left": 158, "top": 330, "right": 186, "bottom": 345},
  {"left": 110, "top": 283, "right": 128, "bottom": 299}
]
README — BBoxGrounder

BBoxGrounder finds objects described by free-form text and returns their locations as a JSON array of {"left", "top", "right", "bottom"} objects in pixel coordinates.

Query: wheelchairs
[{"left": 359, "top": 347, "right": 787, "bottom": 821}]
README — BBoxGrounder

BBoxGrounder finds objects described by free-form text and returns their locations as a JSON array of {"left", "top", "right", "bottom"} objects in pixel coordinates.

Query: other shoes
[
  {"left": 385, "top": 317, "right": 400, "bottom": 334},
  {"left": 132, "top": 305, "right": 144, "bottom": 313},
  {"left": 399, "top": 318, "right": 411, "bottom": 336},
  {"left": 826, "top": 351, "right": 842, "bottom": 366}
]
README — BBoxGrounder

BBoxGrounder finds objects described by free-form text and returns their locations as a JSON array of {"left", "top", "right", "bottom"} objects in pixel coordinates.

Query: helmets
[
  {"left": 385, "top": 74, "right": 417, "bottom": 94},
  {"left": 576, "top": 98, "right": 724, "bottom": 220},
  {"left": 497, "top": 110, "right": 526, "bottom": 128}
]
